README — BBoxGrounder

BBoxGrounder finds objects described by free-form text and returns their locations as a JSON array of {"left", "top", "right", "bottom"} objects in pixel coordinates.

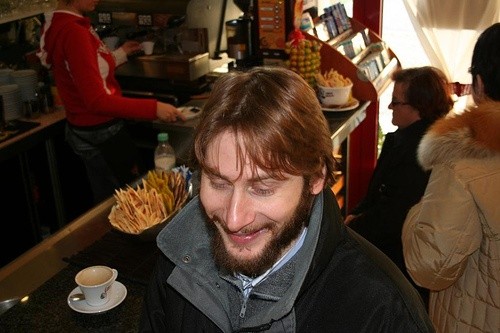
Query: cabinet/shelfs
[{"left": 300, "top": 10, "right": 402, "bottom": 214}]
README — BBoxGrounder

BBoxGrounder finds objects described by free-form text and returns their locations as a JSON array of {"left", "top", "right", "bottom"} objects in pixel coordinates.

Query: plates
[
  {"left": 317, "top": 98, "right": 359, "bottom": 111},
  {"left": 67, "top": 280, "right": 127, "bottom": 313}
]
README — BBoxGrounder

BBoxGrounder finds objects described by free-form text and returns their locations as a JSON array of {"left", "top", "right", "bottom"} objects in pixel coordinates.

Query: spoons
[{"left": 70, "top": 293, "right": 85, "bottom": 302}]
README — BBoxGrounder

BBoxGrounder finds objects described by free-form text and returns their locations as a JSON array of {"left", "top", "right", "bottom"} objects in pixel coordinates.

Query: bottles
[{"left": 155, "top": 133, "right": 176, "bottom": 175}]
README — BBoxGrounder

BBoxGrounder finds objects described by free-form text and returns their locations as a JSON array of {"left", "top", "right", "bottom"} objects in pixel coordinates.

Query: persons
[
  {"left": 344, "top": 66, "right": 455, "bottom": 314},
  {"left": 46, "top": 0, "right": 185, "bottom": 211},
  {"left": 0, "top": 68, "right": 432, "bottom": 333},
  {"left": 400, "top": 23, "right": 500, "bottom": 333}
]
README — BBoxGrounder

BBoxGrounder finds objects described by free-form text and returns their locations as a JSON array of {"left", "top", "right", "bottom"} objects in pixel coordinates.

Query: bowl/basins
[
  {"left": 316, "top": 82, "right": 353, "bottom": 107},
  {"left": 0, "top": 69, "right": 37, "bottom": 121}
]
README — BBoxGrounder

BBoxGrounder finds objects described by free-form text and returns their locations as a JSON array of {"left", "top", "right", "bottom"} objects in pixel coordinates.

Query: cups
[
  {"left": 75, "top": 265, "right": 118, "bottom": 307},
  {"left": 139, "top": 41, "right": 154, "bottom": 55},
  {"left": 103, "top": 37, "right": 118, "bottom": 51}
]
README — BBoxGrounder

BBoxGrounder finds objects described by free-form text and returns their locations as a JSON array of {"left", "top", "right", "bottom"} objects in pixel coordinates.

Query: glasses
[{"left": 392, "top": 98, "right": 409, "bottom": 110}]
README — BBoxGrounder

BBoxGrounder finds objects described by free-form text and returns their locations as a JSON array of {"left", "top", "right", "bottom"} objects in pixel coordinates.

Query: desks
[{"left": 0, "top": 107, "right": 68, "bottom": 232}]
